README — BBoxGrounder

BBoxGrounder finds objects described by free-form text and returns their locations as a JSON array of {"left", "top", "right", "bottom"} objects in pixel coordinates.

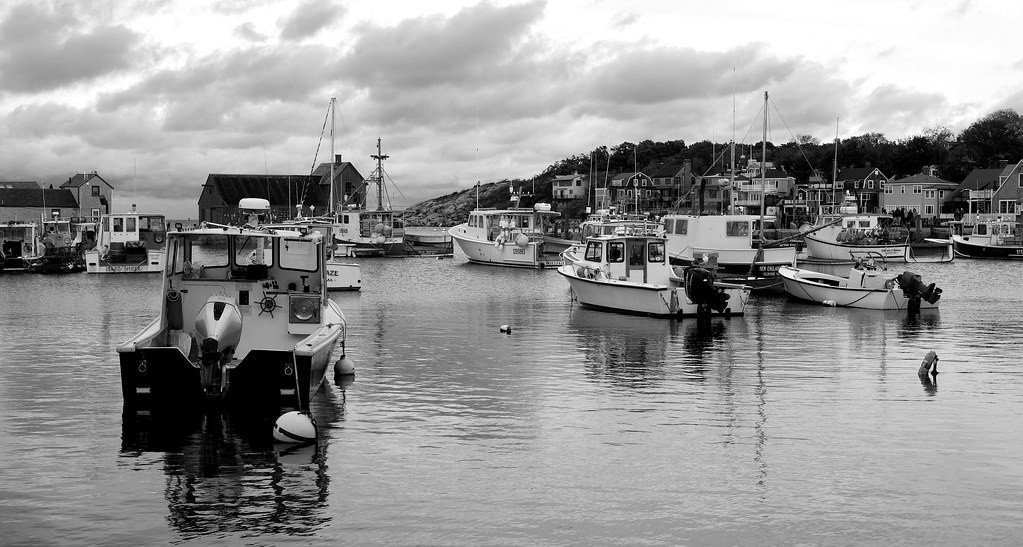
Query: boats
[
  {"left": 115, "top": 198, "right": 354, "bottom": 444},
  {"left": 448, "top": 89, "right": 1023, "bottom": 317},
  {"left": 0, "top": 179, "right": 166, "bottom": 276},
  {"left": 211, "top": 96, "right": 407, "bottom": 291}
]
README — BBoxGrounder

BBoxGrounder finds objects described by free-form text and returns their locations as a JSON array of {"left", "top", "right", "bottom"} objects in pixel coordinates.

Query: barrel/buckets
[{"left": 109, "top": 242, "right": 125, "bottom": 264}]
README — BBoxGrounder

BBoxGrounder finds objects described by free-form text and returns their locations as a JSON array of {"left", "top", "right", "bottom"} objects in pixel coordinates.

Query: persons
[
  {"left": 884, "top": 205, "right": 918, "bottom": 227},
  {"left": 954, "top": 208, "right": 964, "bottom": 235}
]
